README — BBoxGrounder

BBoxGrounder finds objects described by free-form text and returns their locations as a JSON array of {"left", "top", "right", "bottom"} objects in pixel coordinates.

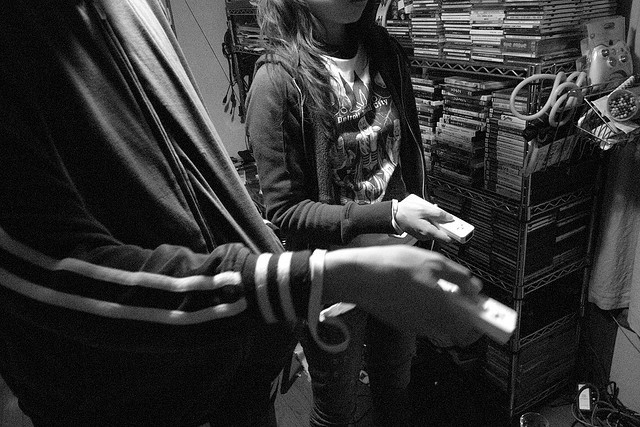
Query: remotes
[{"left": 578, "top": 383, "right": 590, "bottom": 410}]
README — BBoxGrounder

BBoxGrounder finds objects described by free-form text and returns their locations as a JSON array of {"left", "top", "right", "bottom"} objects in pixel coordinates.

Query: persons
[
  {"left": 246, "top": 0, "right": 450, "bottom": 426},
  {"left": 1, "top": 0, "right": 485, "bottom": 427}
]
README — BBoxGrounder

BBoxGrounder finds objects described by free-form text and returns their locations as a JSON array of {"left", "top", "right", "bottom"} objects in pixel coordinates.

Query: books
[
  {"left": 235, "top": 26, "right": 268, "bottom": 52},
  {"left": 385, "top": 1, "right": 625, "bottom": 72},
  {"left": 406, "top": 75, "right": 585, "bottom": 275},
  {"left": 480, "top": 327, "right": 575, "bottom": 408}
]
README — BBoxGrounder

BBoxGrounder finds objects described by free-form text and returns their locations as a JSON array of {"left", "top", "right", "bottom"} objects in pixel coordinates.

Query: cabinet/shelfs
[{"left": 226, "top": 19, "right": 604, "bottom": 416}]
